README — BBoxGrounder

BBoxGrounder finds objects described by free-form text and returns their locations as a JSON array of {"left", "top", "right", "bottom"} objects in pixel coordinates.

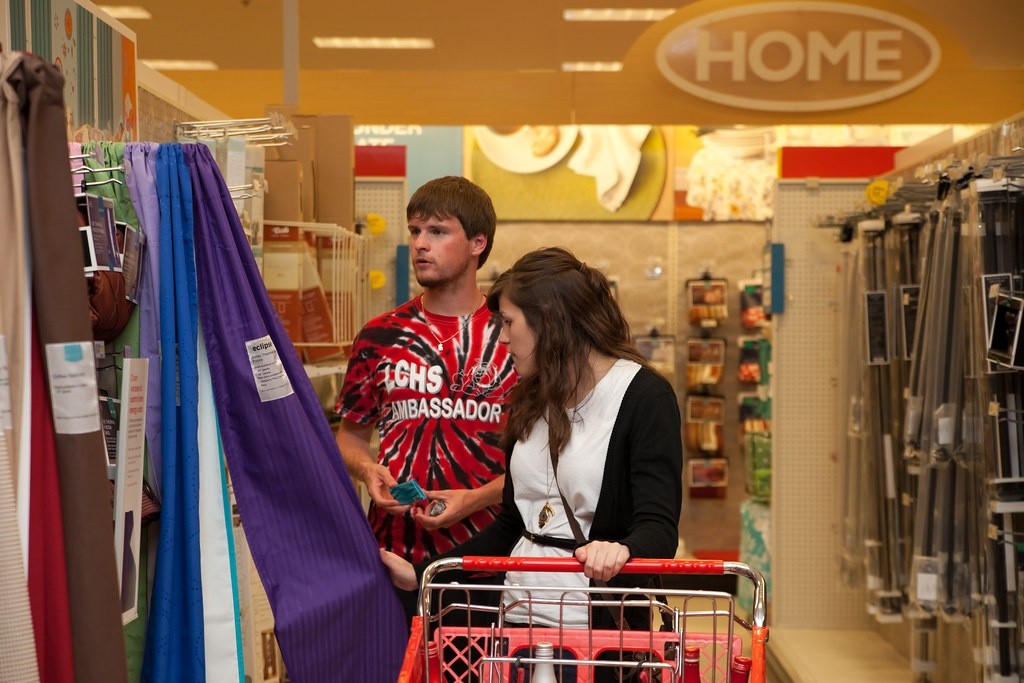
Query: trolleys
[{"left": 397, "top": 554, "right": 768, "bottom": 683}]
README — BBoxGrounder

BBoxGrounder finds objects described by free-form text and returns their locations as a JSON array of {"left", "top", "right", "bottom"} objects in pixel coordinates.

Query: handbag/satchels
[{"left": 597, "top": 578, "right": 678, "bottom": 683}]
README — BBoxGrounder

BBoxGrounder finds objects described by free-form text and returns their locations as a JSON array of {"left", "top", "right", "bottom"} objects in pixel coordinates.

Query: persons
[
  {"left": 334, "top": 175, "right": 523, "bottom": 683},
  {"left": 487, "top": 246, "right": 684, "bottom": 683}
]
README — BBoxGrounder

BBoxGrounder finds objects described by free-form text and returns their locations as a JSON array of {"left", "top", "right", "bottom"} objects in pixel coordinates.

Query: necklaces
[
  {"left": 538, "top": 411, "right": 573, "bottom": 530},
  {"left": 421, "top": 295, "right": 482, "bottom": 351}
]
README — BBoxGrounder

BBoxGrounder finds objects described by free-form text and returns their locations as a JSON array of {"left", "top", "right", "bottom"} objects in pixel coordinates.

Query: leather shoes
[{"left": 523, "top": 529, "right": 587, "bottom": 550}]
